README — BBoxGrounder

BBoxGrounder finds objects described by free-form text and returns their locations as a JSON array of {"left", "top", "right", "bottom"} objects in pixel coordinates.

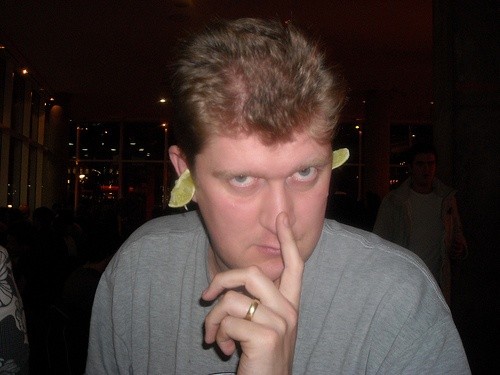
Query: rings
[{"left": 245, "top": 299, "right": 260, "bottom": 321}]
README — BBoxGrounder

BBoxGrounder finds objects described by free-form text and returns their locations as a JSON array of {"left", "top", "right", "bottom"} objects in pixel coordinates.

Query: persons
[
  {"left": 0, "top": 206, "right": 131, "bottom": 375},
  {"left": 373, "top": 142, "right": 470, "bottom": 309},
  {"left": 84, "top": 18, "right": 471, "bottom": 375}
]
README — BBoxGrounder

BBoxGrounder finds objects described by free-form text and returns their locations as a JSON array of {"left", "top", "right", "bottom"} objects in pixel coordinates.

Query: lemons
[
  {"left": 332, "top": 148, "right": 350, "bottom": 169},
  {"left": 168, "top": 168, "right": 195, "bottom": 208}
]
